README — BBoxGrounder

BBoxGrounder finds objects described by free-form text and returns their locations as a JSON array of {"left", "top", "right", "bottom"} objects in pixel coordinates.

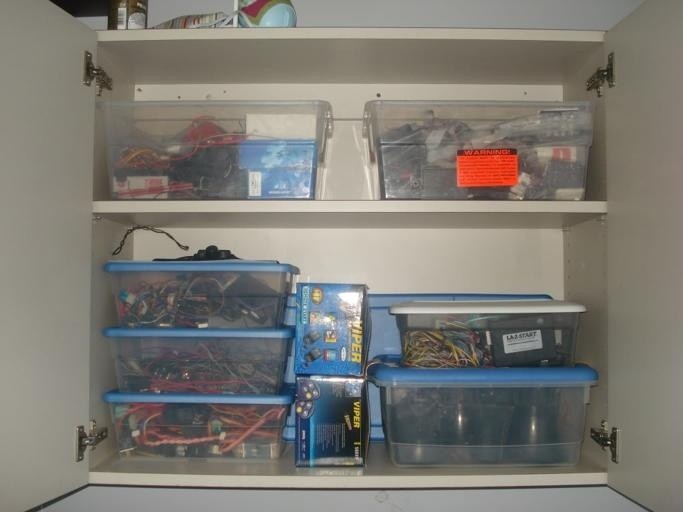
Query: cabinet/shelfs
[{"left": 1, "top": 3, "right": 681, "bottom": 512}]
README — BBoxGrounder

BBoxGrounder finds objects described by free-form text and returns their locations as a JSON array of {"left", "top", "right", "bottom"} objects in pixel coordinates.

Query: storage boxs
[
  {"left": 94, "top": 99, "right": 333, "bottom": 200},
  {"left": 91, "top": 262, "right": 600, "bottom": 471},
  {"left": 362, "top": 99, "right": 595, "bottom": 209}
]
